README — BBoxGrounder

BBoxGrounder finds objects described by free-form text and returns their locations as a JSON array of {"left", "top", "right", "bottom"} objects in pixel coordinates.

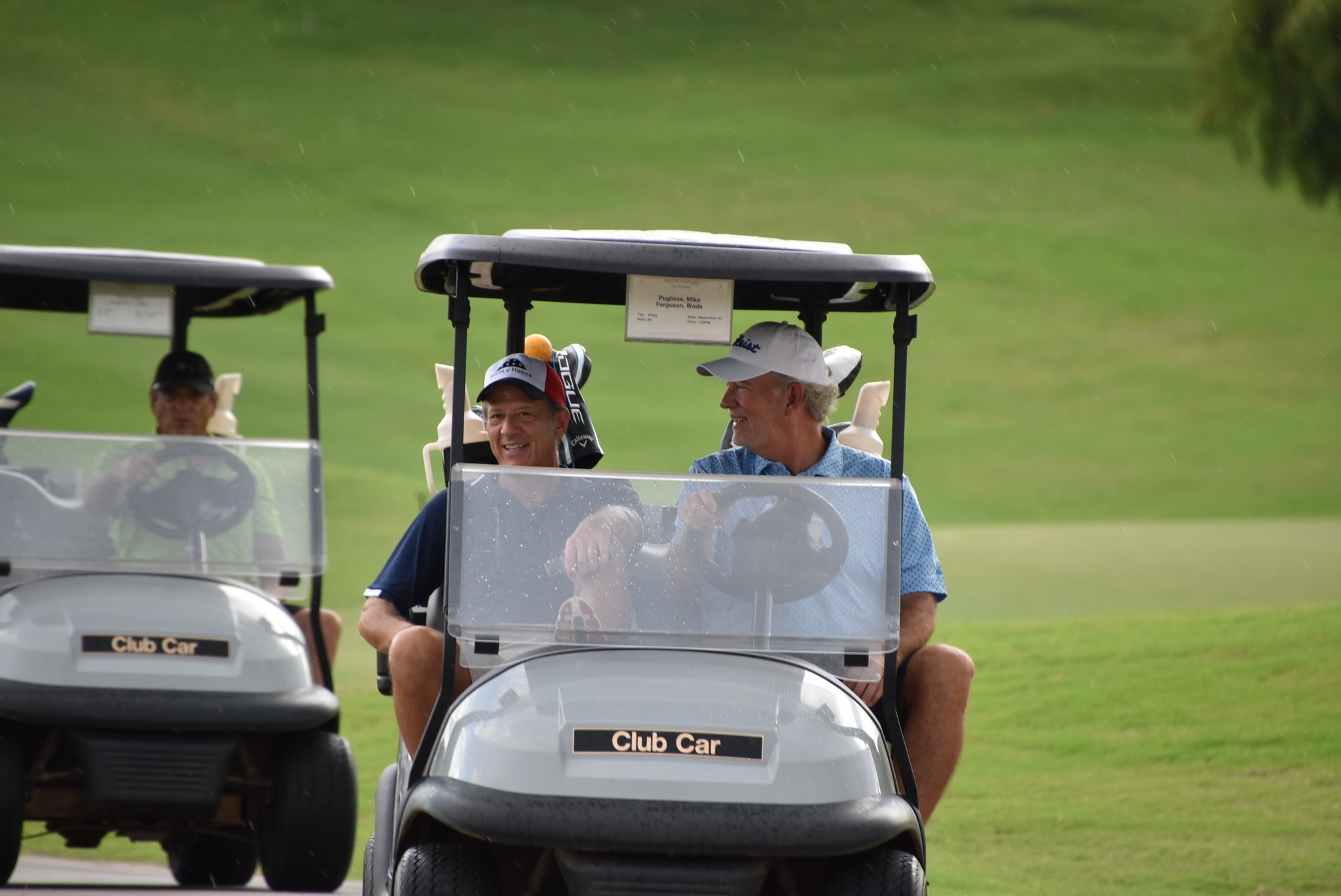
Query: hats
[
  {"left": 477, "top": 353, "right": 565, "bottom": 410},
  {"left": 696, "top": 322, "right": 827, "bottom": 387},
  {"left": 153, "top": 351, "right": 213, "bottom": 395}
]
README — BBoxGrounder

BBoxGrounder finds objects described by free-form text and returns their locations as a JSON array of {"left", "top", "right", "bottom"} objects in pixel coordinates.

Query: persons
[
  {"left": 360, "top": 353, "right": 647, "bottom": 764},
  {"left": 668, "top": 322, "right": 973, "bottom": 832},
  {"left": 86, "top": 354, "right": 341, "bottom": 684}
]
